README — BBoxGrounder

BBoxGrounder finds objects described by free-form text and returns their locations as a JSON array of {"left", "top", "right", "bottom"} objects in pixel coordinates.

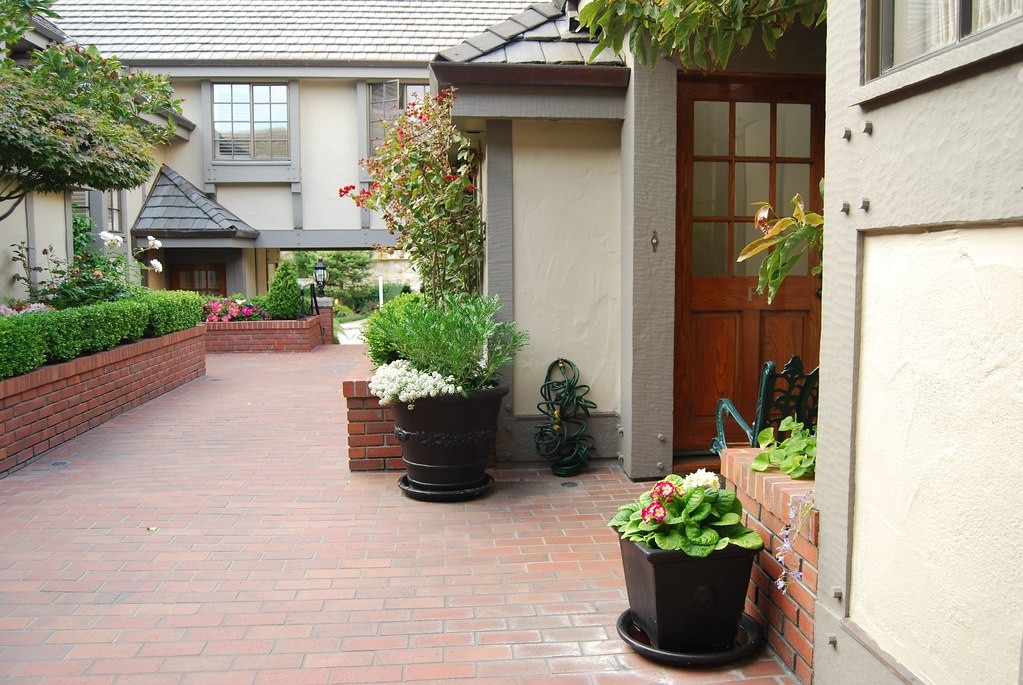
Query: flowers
[
  {"left": 606, "top": 468, "right": 765, "bottom": 557},
  {"left": 355, "top": 290, "right": 531, "bottom": 410}
]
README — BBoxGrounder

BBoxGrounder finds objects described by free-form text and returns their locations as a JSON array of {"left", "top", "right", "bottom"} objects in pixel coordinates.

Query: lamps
[{"left": 313, "top": 257, "right": 330, "bottom": 299}]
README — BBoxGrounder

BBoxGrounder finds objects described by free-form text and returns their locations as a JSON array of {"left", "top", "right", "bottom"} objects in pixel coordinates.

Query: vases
[
  {"left": 390, "top": 379, "right": 509, "bottom": 504},
  {"left": 611, "top": 525, "right": 765, "bottom": 671}
]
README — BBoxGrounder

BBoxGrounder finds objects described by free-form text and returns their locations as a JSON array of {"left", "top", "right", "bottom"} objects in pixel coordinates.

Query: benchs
[{"left": 709, "top": 352, "right": 819, "bottom": 459}]
